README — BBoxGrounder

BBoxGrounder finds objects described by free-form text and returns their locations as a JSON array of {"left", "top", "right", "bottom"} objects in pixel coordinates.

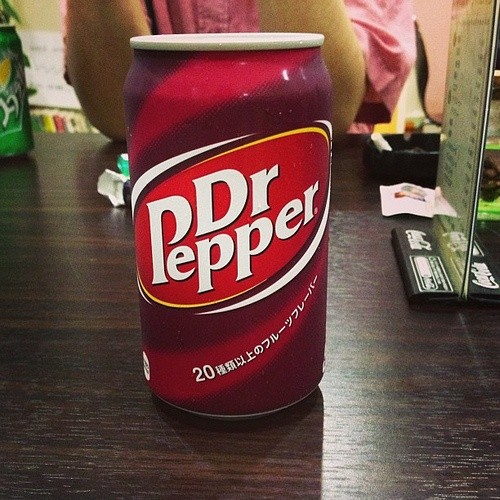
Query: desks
[{"left": 0, "top": 133, "right": 500, "bottom": 500}]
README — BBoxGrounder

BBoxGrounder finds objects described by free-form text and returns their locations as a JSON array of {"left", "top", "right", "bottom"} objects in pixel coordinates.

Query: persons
[{"left": 61, "top": 0, "right": 418, "bottom": 146}]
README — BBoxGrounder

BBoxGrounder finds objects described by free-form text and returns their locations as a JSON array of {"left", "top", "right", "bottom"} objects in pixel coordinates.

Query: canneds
[
  {"left": 0, "top": 25, "right": 33, "bottom": 160},
  {"left": 122, "top": 31, "right": 334, "bottom": 420}
]
